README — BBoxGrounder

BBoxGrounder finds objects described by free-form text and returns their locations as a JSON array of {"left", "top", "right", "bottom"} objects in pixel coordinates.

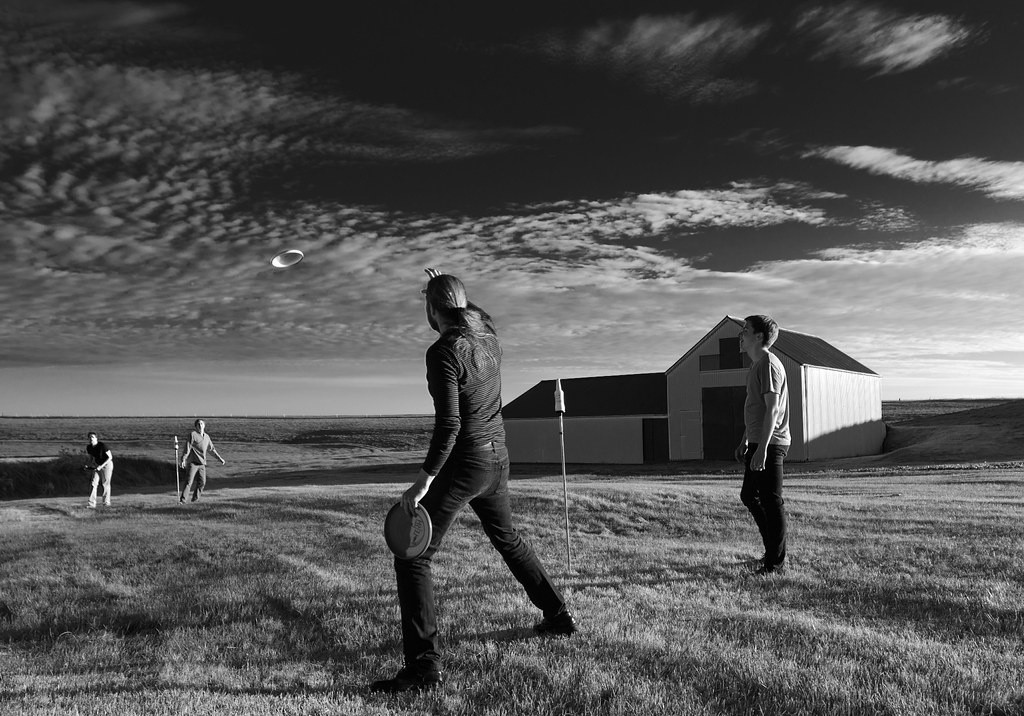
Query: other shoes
[
  {"left": 745, "top": 558, "right": 785, "bottom": 573},
  {"left": 86, "top": 505, "right": 95, "bottom": 509},
  {"left": 104, "top": 503, "right": 110, "bottom": 507},
  {"left": 178, "top": 501, "right": 185, "bottom": 505}
]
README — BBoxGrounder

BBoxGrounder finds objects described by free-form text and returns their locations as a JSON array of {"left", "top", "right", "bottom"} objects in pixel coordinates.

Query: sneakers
[
  {"left": 370, "top": 665, "right": 443, "bottom": 691},
  {"left": 533, "top": 610, "right": 579, "bottom": 633}
]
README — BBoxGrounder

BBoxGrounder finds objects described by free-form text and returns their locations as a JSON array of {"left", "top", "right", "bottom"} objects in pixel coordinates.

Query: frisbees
[
  {"left": 270, "top": 248, "right": 305, "bottom": 268},
  {"left": 384, "top": 502, "right": 432, "bottom": 559}
]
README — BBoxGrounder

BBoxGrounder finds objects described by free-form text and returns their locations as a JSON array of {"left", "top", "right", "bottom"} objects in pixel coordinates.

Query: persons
[
  {"left": 179, "top": 419, "right": 226, "bottom": 504},
  {"left": 734, "top": 316, "right": 792, "bottom": 573},
  {"left": 84, "top": 432, "right": 114, "bottom": 508},
  {"left": 369, "top": 268, "right": 577, "bottom": 692}
]
